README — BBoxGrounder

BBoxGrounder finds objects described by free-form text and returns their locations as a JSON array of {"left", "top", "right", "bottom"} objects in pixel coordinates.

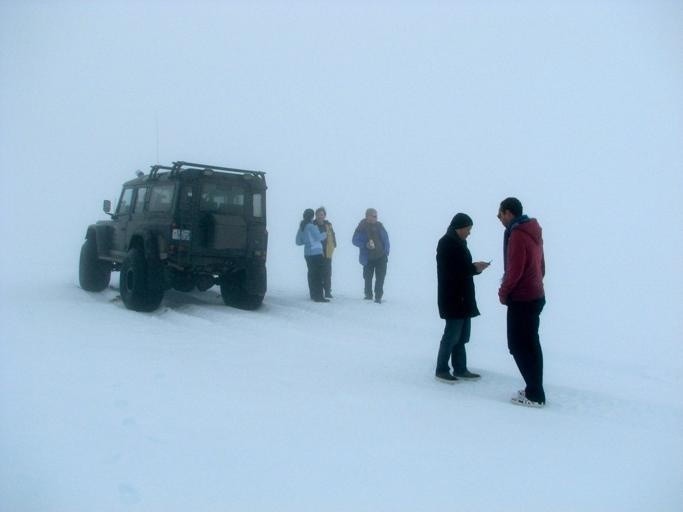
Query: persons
[
  {"left": 351, "top": 208, "right": 389, "bottom": 304},
  {"left": 433, "top": 212, "right": 491, "bottom": 384},
  {"left": 312, "top": 206, "right": 336, "bottom": 298},
  {"left": 295, "top": 208, "right": 330, "bottom": 302},
  {"left": 496, "top": 197, "right": 547, "bottom": 408}
]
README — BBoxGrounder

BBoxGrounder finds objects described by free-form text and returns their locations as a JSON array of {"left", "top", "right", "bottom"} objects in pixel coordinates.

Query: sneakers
[
  {"left": 452, "top": 368, "right": 480, "bottom": 380},
  {"left": 310, "top": 293, "right": 382, "bottom": 304},
  {"left": 510, "top": 389, "right": 545, "bottom": 408},
  {"left": 435, "top": 371, "right": 459, "bottom": 382}
]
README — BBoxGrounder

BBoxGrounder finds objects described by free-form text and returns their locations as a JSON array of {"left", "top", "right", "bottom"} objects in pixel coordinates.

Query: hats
[{"left": 449, "top": 212, "right": 473, "bottom": 229}]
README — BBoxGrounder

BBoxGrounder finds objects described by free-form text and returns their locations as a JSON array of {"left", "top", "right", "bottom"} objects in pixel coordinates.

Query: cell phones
[{"left": 486, "top": 257, "right": 494, "bottom": 266}]
